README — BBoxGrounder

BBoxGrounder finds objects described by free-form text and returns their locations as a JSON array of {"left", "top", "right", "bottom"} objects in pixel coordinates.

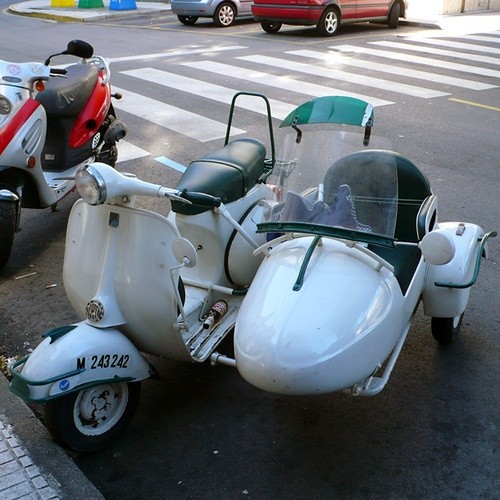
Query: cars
[
  {"left": 170, "top": 0, "right": 258, "bottom": 27},
  {"left": 250, "top": 0, "right": 408, "bottom": 38}
]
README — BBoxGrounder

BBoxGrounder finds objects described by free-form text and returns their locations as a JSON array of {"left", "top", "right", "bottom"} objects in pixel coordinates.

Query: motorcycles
[
  {"left": 0, "top": 41, "right": 125, "bottom": 262},
  {"left": 5, "top": 94, "right": 496, "bottom": 454}
]
